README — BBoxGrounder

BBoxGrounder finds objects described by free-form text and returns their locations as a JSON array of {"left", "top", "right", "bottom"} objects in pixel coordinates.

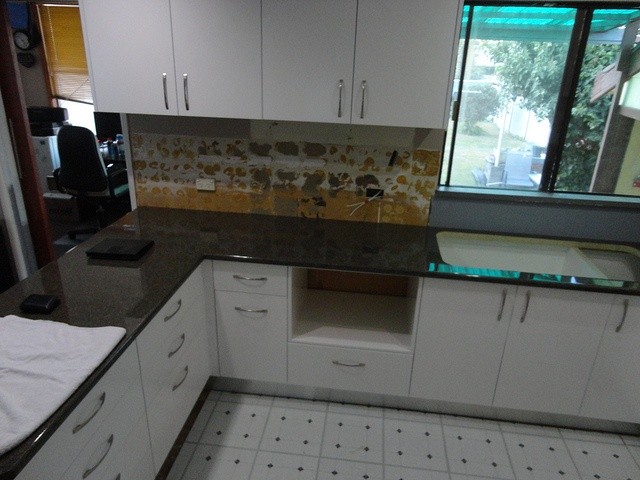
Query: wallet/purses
[{"left": 21, "top": 293, "right": 59, "bottom": 313}]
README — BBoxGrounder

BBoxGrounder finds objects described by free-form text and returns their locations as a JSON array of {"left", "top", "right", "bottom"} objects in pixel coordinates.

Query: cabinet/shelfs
[
  {"left": 16, "top": 339, "right": 157, "bottom": 480},
  {"left": 579, "top": 294, "right": 639, "bottom": 425},
  {"left": 78, "top": 0, "right": 262, "bottom": 120},
  {"left": 410, "top": 277, "right": 615, "bottom": 417},
  {"left": 211, "top": 260, "right": 308, "bottom": 384},
  {"left": 288, "top": 340, "right": 412, "bottom": 398},
  {"left": 262, "top": 0, "right": 463, "bottom": 132},
  {"left": 136, "top": 264, "right": 211, "bottom": 476}
]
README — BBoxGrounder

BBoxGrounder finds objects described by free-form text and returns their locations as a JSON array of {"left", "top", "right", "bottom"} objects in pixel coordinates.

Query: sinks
[
  {"left": 437, "top": 231, "right": 603, "bottom": 279},
  {"left": 580, "top": 244, "right": 640, "bottom": 282}
]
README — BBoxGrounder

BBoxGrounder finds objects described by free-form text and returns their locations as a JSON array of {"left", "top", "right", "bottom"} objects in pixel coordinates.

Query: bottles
[{"left": 107, "top": 137, "right": 114, "bottom": 156}]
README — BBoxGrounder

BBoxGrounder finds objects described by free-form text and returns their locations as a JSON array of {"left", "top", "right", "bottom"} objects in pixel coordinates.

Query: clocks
[{"left": 13, "top": 30, "right": 40, "bottom": 51}]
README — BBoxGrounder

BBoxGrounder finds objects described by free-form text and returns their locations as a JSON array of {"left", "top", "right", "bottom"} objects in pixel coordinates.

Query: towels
[{"left": 0, "top": 314, "right": 126, "bottom": 454}]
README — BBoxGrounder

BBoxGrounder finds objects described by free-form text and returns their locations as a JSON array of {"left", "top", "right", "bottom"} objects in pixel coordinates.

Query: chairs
[{"left": 52, "top": 124, "right": 125, "bottom": 243}]
